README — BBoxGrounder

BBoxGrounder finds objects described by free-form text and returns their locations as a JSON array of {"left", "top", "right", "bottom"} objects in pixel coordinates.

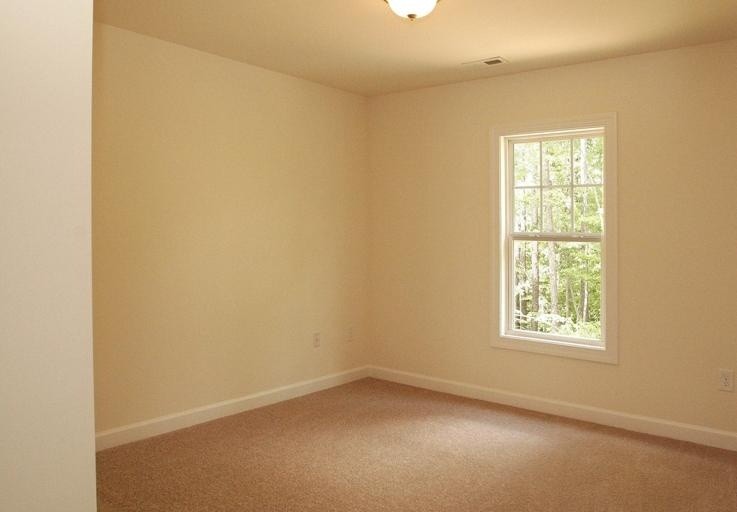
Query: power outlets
[{"left": 717, "top": 367, "right": 735, "bottom": 392}]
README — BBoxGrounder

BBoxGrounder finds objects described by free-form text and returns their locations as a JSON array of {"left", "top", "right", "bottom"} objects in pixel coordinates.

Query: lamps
[{"left": 384, "top": 0, "right": 441, "bottom": 20}]
